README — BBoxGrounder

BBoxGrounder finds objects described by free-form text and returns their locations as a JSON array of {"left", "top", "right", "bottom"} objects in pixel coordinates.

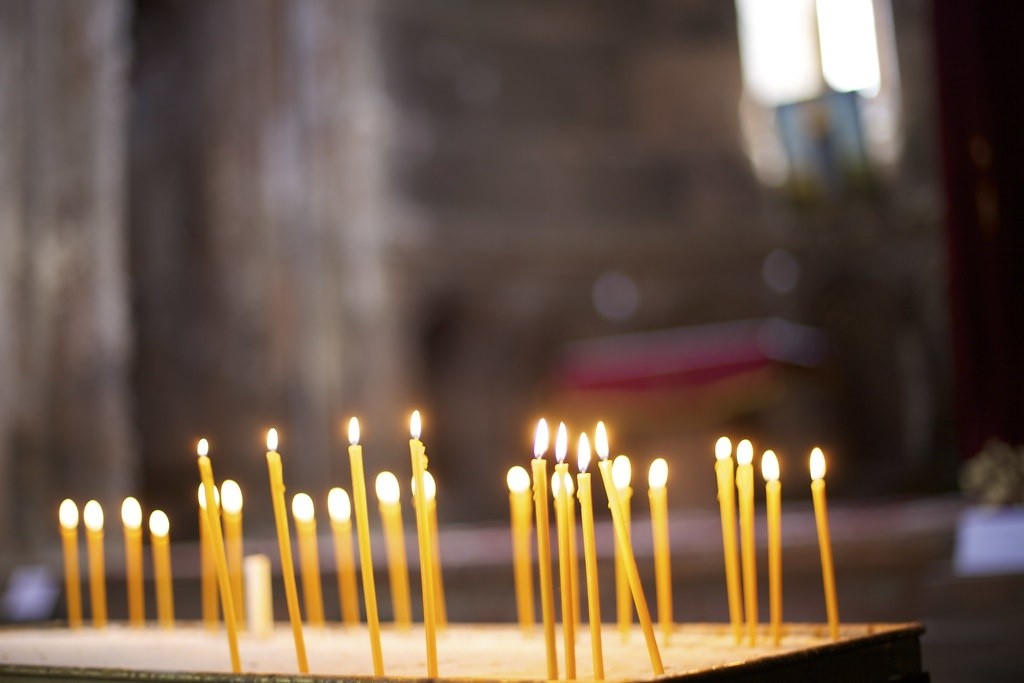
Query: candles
[
  {"left": 734, "top": 438, "right": 762, "bottom": 647},
  {"left": 196, "top": 437, "right": 242, "bottom": 674},
  {"left": 148, "top": 509, "right": 176, "bottom": 632},
  {"left": 327, "top": 487, "right": 362, "bottom": 628},
  {"left": 293, "top": 491, "right": 328, "bottom": 627},
  {"left": 347, "top": 416, "right": 385, "bottom": 679},
  {"left": 122, "top": 497, "right": 148, "bottom": 631},
  {"left": 265, "top": 428, "right": 310, "bottom": 676},
  {"left": 712, "top": 437, "right": 746, "bottom": 644},
  {"left": 82, "top": 499, "right": 108, "bottom": 633},
  {"left": 645, "top": 457, "right": 673, "bottom": 640},
  {"left": 198, "top": 481, "right": 220, "bottom": 630},
  {"left": 59, "top": 499, "right": 84, "bottom": 631},
  {"left": 376, "top": 470, "right": 416, "bottom": 639},
  {"left": 506, "top": 466, "right": 538, "bottom": 642},
  {"left": 809, "top": 447, "right": 842, "bottom": 641},
  {"left": 221, "top": 477, "right": 245, "bottom": 629},
  {"left": 758, "top": 451, "right": 784, "bottom": 646},
  {"left": 406, "top": 411, "right": 447, "bottom": 678},
  {"left": 531, "top": 416, "right": 665, "bottom": 682}
]
